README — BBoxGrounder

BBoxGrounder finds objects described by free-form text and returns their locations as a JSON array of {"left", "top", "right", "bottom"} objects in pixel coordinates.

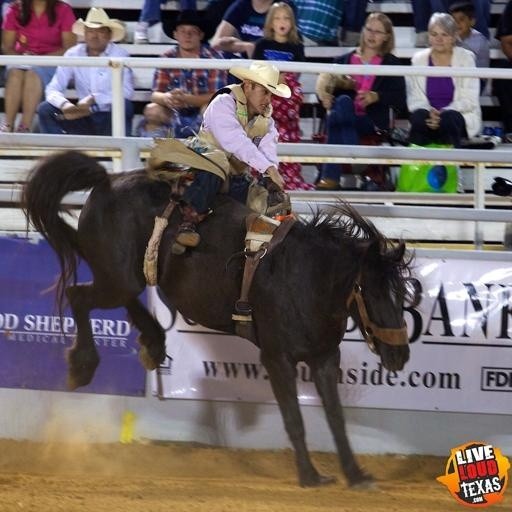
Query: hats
[
  {"left": 163, "top": 9, "right": 216, "bottom": 43},
  {"left": 69, "top": 6, "right": 127, "bottom": 43},
  {"left": 228, "top": 60, "right": 294, "bottom": 99}
]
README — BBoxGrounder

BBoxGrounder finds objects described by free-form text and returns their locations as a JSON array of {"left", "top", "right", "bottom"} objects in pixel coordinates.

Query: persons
[
  {"left": 403, "top": 11, "right": 483, "bottom": 144},
  {"left": 445, "top": 2, "right": 490, "bottom": 96},
  {"left": 410, "top": 0, "right": 491, "bottom": 48},
  {"left": 33, "top": 5, "right": 135, "bottom": 135},
  {"left": 130, "top": 0, "right": 198, "bottom": 43},
  {"left": 251, "top": 0, "right": 310, "bottom": 61},
  {"left": 209, "top": 0, "right": 298, "bottom": 59},
  {"left": 143, "top": 9, "right": 231, "bottom": 138},
  {"left": 289, "top": 0, "right": 345, "bottom": 63},
  {"left": 1, "top": 0, "right": 78, "bottom": 134},
  {"left": 491, "top": 0, "right": 511, "bottom": 143},
  {"left": 313, "top": 11, "right": 408, "bottom": 191},
  {"left": 147, "top": 55, "right": 293, "bottom": 257}
]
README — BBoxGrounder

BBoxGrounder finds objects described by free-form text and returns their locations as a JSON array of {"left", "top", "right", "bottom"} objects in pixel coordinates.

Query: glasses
[{"left": 364, "top": 28, "right": 388, "bottom": 36}]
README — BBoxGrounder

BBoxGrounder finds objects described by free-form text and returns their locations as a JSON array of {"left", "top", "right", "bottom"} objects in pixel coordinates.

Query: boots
[{"left": 170, "top": 221, "right": 200, "bottom": 256}]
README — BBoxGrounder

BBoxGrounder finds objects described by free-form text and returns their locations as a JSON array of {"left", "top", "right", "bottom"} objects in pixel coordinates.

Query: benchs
[
  {"left": 0, "top": 1, "right": 506, "bottom": 62},
  {"left": 1, "top": 44, "right": 499, "bottom": 146}
]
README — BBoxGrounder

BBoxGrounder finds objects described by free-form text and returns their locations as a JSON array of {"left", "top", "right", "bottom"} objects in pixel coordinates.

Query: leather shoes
[{"left": 317, "top": 175, "right": 339, "bottom": 190}]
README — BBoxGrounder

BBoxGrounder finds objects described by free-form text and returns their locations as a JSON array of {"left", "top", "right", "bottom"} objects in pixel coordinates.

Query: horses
[{"left": 18, "top": 149, "right": 423, "bottom": 491}]
